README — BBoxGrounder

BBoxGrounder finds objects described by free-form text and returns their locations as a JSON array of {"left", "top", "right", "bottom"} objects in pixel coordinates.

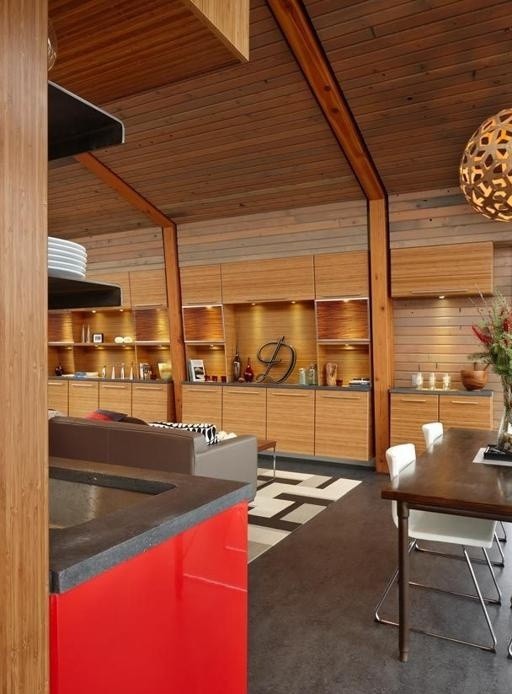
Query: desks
[{"left": 369, "top": 424, "right": 511, "bottom": 665}]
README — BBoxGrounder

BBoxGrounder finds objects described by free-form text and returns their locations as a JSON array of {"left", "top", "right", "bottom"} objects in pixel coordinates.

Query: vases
[{"left": 491, "top": 371, "right": 511, "bottom": 457}]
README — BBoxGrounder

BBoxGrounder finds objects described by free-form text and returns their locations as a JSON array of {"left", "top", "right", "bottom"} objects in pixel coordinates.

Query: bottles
[
  {"left": 244, "top": 356, "right": 253, "bottom": 382},
  {"left": 102, "top": 367, "right": 106, "bottom": 378},
  {"left": 121, "top": 365, "right": 125, "bottom": 378},
  {"left": 429, "top": 372, "right": 436, "bottom": 389},
  {"left": 443, "top": 372, "right": 451, "bottom": 389},
  {"left": 415, "top": 371, "right": 424, "bottom": 389},
  {"left": 130, "top": 366, "right": 134, "bottom": 379},
  {"left": 299, "top": 363, "right": 318, "bottom": 386},
  {"left": 112, "top": 366, "right": 116, "bottom": 379}
]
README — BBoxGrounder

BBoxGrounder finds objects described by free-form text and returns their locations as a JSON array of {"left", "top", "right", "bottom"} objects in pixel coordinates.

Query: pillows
[{"left": 47, "top": 405, "right": 239, "bottom": 447}]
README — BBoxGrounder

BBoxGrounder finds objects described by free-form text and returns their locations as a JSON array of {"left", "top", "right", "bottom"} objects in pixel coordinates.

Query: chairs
[
  {"left": 409, "top": 421, "right": 509, "bottom": 570},
  {"left": 372, "top": 439, "right": 506, "bottom": 654}
]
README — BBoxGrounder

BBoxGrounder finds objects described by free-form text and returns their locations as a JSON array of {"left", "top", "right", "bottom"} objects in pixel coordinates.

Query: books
[
  {"left": 139, "top": 362, "right": 151, "bottom": 380},
  {"left": 188, "top": 358, "right": 206, "bottom": 382}
]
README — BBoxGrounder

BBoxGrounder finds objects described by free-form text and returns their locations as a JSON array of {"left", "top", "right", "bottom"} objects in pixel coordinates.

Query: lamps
[{"left": 455, "top": 107, "right": 512, "bottom": 226}]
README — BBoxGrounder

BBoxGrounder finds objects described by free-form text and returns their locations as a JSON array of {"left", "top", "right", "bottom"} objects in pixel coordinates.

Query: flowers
[{"left": 458, "top": 276, "right": 512, "bottom": 454}]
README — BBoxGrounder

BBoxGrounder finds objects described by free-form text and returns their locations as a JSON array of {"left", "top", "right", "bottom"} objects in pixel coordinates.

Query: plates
[{"left": 48, "top": 235, "right": 88, "bottom": 278}]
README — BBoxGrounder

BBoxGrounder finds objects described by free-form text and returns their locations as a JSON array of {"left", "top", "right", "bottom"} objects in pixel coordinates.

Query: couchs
[{"left": 48, "top": 408, "right": 260, "bottom": 511}]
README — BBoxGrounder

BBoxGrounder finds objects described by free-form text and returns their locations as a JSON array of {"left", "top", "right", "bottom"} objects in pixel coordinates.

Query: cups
[{"left": 203, "top": 374, "right": 227, "bottom": 384}]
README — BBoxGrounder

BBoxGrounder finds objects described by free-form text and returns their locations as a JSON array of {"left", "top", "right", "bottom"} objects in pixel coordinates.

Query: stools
[{"left": 254, "top": 437, "right": 277, "bottom": 493}]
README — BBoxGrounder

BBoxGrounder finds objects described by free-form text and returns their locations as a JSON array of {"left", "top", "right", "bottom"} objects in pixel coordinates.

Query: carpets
[{"left": 235, "top": 465, "right": 365, "bottom": 569}]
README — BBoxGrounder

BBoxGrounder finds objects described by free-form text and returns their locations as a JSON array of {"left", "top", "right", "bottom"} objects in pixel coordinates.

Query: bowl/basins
[{"left": 460, "top": 369, "right": 489, "bottom": 389}]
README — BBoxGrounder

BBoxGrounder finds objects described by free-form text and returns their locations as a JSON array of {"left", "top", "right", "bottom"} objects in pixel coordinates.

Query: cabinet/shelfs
[
  {"left": 43, "top": 70, "right": 130, "bottom": 316},
  {"left": 40, "top": 2, "right": 256, "bottom": 112}
]
